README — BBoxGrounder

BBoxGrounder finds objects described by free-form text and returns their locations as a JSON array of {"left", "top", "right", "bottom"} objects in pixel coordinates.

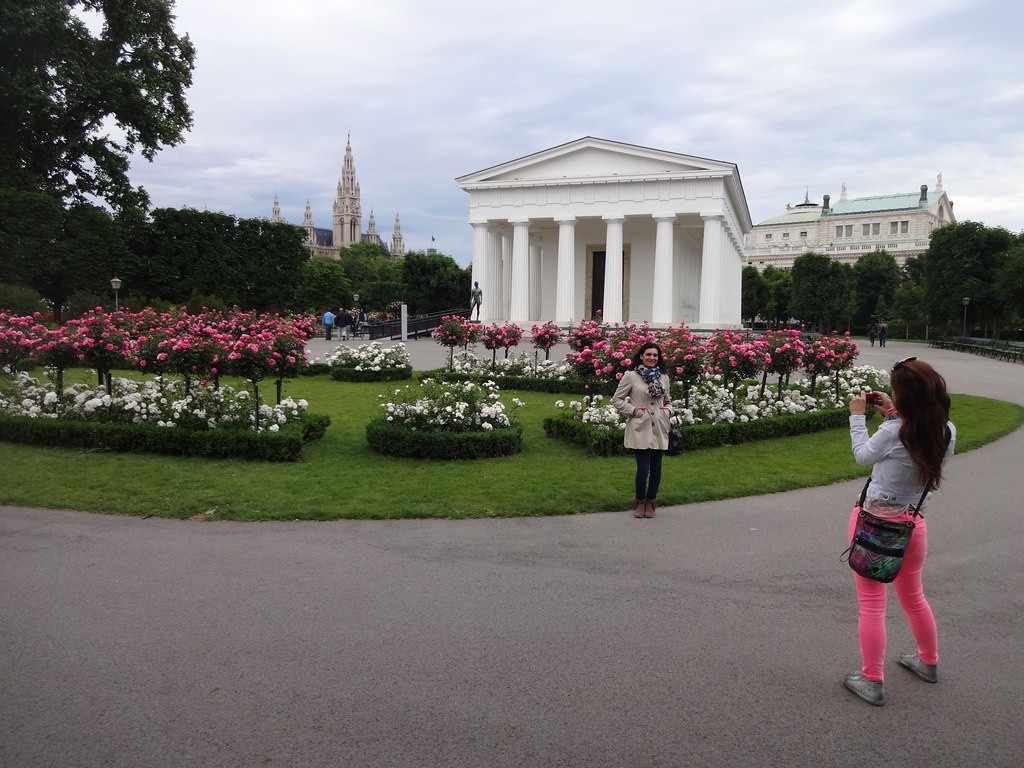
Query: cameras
[{"left": 865, "top": 393, "right": 878, "bottom": 404}]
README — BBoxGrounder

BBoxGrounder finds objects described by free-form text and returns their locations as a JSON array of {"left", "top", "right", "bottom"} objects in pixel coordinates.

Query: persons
[
  {"left": 868, "top": 319, "right": 888, "bottom": 347},
  {"left": 848, "top": 355, "right": 957, "bottom": 707},
  {"left": 611, "top": 342, "right": 674, "bottom": 519},
  {"left": 467, "top": 282, "right": 483, "bottom": 321},
  {"left": 321, "top": 308, "right": 367, "bottom": 341}
]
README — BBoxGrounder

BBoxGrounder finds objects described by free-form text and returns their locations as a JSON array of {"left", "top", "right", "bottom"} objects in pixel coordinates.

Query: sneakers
[
  {"left": 844, "top": 671, "right": 886, "bottom": 707},
  {"left": 897, "top": 653, "right": 939, "bottom": 683}
]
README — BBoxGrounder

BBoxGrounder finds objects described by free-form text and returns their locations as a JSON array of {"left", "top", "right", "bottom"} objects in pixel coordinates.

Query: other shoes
[
  {"left": 347, "top": 338, "right": 349, "bottom": 340},
  {"left": 342, "top": 336, "right": 345, "bottom": 340}
]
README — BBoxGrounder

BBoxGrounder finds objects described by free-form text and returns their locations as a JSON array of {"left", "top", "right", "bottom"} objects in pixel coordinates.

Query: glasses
[{"left": 892, "top": 356, "right": 918, "bottom": 369}]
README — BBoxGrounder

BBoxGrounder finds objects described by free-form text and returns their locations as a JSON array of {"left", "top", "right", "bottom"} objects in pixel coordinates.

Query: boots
[
  {"left": 645, "top": 499, "right": 655, "bottom": 517},
  {"left": 634, "top": 499, "right": 646, "bottom": 518}
]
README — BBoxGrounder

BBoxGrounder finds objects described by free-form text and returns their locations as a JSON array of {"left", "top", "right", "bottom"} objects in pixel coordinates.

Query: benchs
[
  {"left": 928, "top": 335, "right": 1024, "bottom": 363},
  {"left": 353, "top": 326, "right": 371, "bottom": 340}
]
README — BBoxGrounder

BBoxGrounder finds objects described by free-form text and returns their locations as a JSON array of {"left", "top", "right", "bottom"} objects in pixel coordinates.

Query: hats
[{"left": 339, "top": 308, "right": 343, "bottom": 311}]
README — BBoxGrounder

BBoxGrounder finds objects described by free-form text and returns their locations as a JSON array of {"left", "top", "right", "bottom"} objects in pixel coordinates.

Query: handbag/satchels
[{"left": 840, "top": 509, "right": 917, "bottom": 585}]
